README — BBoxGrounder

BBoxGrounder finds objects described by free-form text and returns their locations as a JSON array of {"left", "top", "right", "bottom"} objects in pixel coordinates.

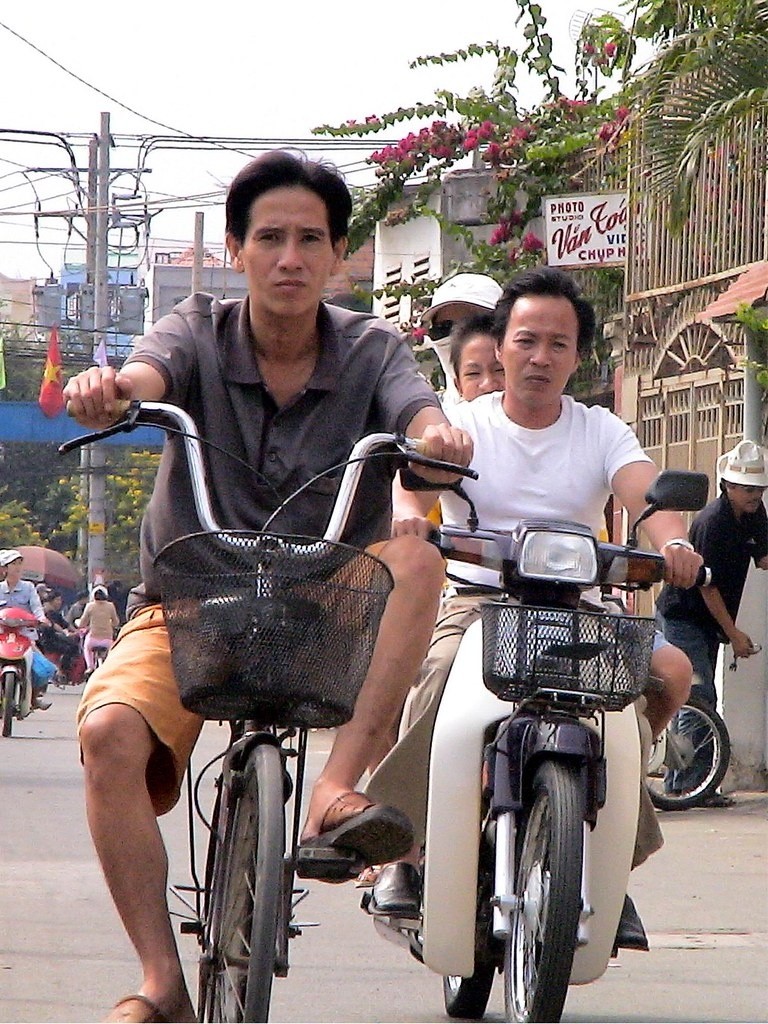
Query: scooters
[{"left": 354, "top": 466, "right": 716, "bottom": 1024}]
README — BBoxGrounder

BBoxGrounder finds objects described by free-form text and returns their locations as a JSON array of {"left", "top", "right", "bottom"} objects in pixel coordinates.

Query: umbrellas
[{"left": 9, "top": 545, "right": 82, "bottom": 589}]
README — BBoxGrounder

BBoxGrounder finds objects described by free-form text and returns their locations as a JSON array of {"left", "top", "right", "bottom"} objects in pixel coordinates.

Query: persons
[
  {"left": 63, "top": 149, "right": 476, "bottom": 1023},
  {"left": 0, "top": 550, "right": 135, "bottom": 712},
  {"left": 653, "top": 439, "right": 768, "bottom": 806},
  {"left": 363, "top": 273, "right": 704, "bottom": 1022}
]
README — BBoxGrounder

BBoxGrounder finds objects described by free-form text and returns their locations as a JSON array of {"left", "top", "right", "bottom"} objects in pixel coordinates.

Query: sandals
[{"left": 355, "top": 866, "right": 379, "bottom": 891}]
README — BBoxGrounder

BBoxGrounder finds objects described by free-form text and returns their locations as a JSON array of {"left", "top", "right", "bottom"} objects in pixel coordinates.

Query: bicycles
[
  {"left": 85, "top": 646, "right": 107, "bottom": 683},
  {"left": 60, "top": 393, "right": 484, "bottom": 1022}
]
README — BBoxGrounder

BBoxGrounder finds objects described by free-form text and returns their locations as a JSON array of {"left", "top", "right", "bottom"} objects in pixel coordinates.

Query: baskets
[
  {"left": 477, "top": 600, "right": 657, "bottom": 713},
  {"left": 152, "top": 528, "right": 395, "bottom": 732}
]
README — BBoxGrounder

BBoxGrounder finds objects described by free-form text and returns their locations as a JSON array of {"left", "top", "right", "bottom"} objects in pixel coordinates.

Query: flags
[{"left": 38, "top": 325, "right": 64, "bottom": 418}]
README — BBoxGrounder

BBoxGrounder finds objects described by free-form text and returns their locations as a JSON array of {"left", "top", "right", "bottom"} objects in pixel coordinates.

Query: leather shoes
[
  {"left": 612, "top": 893, "right": 650, "bottom": 954},
  {"left": 372, "top": 863, "right": 420, "bottom": 912}
]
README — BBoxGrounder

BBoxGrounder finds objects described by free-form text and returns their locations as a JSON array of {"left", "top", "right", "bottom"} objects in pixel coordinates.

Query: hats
[
  {"left": 38, "top": 582, "right": 52, "bottom": 592},
  {"left": 0, "top": 549, "right": 24, "bottom": 567},
  {"left": 716, "top": 439, "right": 768, "bottom": 487},
  {"left": 92, "top": 585, "right": 108, "bottom": 598},
  {"left": 419, "top": 273, "right": 503, "bottom": 322}
]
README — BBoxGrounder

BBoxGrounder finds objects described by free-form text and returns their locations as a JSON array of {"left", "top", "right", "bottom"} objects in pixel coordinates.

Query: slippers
[
  {"left": 301, "top": 791, "right": 412, "bottom": 884},
  {"left": 100, "top": 981, "right": 195, "bottom": 1021}
]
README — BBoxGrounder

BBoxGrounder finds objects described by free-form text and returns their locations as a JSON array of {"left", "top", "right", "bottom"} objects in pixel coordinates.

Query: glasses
[
  {"left": 731, "top": 485, "right": 765, "bottom": 494},
  {"left": 428, "top": 315, "right": 496, "bottom": 341}
]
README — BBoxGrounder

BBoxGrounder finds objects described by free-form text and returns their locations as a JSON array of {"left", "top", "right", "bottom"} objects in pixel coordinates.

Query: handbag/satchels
[{"left": 31, "top": 653, "right": 57, "bottom": 687}]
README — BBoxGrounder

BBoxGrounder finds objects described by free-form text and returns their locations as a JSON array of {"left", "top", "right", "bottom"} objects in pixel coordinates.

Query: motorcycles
[
  {"left": 646, "top": 695, "right": 731, "bottom": 812},
  {"left": 0, "top": 597, "right": 54, "bottom": 737}
]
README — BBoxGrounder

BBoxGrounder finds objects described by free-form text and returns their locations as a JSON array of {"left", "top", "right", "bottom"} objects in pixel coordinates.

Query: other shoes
[
  {"left": 706, "top": 793, "right": 736, "bottom": 807},
  {"left": 31, "top": 697, "right": 52, "bottom": 711}
]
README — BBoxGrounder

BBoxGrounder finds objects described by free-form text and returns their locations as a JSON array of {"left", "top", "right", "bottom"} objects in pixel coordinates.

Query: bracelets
[{"left": 663, "top": 537, "right": 693, "bottom": 553}]
